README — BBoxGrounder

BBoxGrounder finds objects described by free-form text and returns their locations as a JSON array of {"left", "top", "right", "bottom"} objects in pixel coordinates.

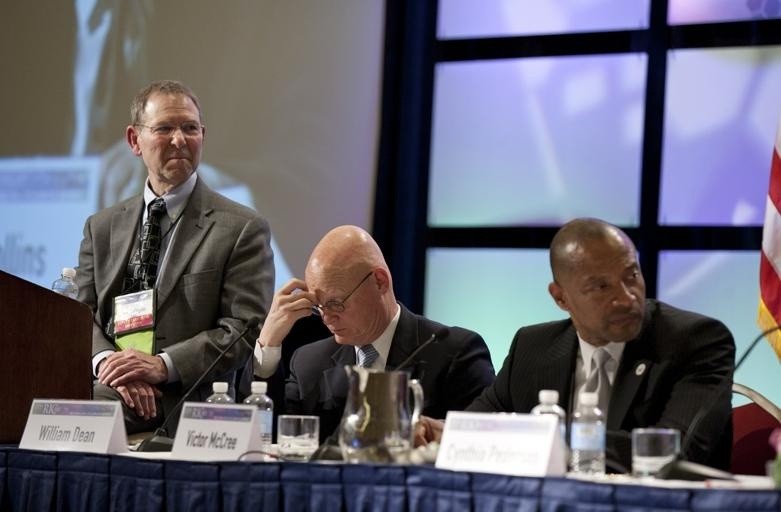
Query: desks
[{"left": 0, "top": 445, "right": 781, "bottom": 512}]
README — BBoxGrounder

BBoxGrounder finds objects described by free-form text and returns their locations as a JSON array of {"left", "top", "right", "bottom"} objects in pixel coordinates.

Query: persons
[
  {"left": 66, "top": 79, "right": 276, "bottom": 437},
  {"left": 409, "top": 216, "right": 736, "bottom": 472},
  {"left": 245, "top": 222, "right": 493, "bottom": 438}
]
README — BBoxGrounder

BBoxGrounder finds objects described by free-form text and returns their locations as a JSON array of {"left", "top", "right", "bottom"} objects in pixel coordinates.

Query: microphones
[
  {"left": 138, "top": 316, "right": 259, "bottom": 452},
  {"left": 310, "top": 326, "right": 449, "bottom": 460},
  {"left": 655, "top": 325, "right": 781, "bottom": 480}
]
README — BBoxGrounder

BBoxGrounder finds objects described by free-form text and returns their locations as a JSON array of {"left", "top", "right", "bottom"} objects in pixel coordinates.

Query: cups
[
  {"left": 277, "top": 414, "right": 320, "bottom": 463},
  {"left": 633, "top": 427, "right": 681, "bottom": 476}
]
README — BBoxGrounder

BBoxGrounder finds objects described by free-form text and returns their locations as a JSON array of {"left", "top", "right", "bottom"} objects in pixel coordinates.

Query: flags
[{"left": 754, "top": 124, "right": 781, "bottom": 364}]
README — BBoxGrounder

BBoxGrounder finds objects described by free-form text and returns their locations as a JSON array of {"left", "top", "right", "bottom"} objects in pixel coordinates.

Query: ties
[
  {"left": 356, "top": 343, "right": 380, "bottom": 369},
  {"left": 577, "top": 348, "right": 611, "bottom": 427},
  {"left": 103, "top": 200, "right": 167, "bottom": 345}
]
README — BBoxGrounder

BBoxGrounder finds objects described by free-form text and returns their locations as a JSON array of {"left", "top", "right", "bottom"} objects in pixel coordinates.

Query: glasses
[
  {"left": 133, "top": 120, "right": 204, "bottom": 139},
  {"left": 311, "top": 270, "right": 377, "bottom": 316}
]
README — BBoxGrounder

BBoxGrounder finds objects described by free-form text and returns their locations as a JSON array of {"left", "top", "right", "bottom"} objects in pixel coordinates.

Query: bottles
[
  {"left": 531, "top": 389, "right": 608, "bottom": 475},
  {"left": 206, "top": 381, "right": 274, "bottom": 464},
  {"left": 53, "top": 268, "right": 79, "bottom": 301}
]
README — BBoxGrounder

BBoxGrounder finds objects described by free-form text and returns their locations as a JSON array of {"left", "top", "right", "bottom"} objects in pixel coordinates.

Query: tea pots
[{"left": 339, "top": 363, "right": 424, "bottom": 465}]
